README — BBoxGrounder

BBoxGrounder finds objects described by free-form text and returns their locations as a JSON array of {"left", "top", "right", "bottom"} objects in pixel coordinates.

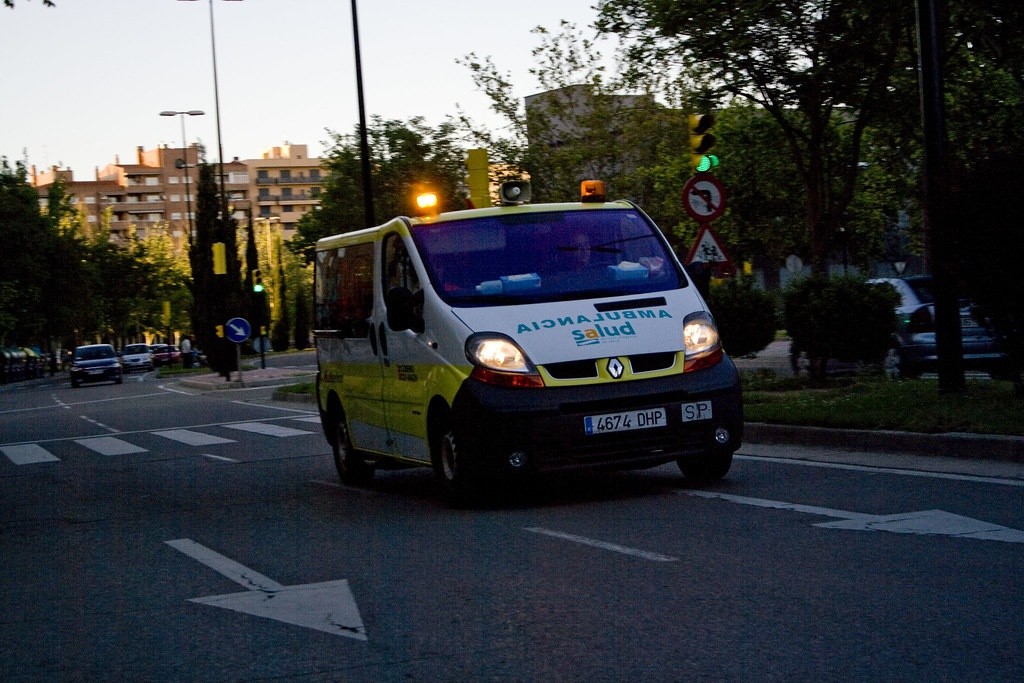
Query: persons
[
  {"left": 412, "top": 240, "right": 475, "bottom": 299},
  {"left": 549, "top": 228, "right": 622, "bottom": 297},
  {"left": 181, "top": 335, "right": 194, "bottom": 368}
]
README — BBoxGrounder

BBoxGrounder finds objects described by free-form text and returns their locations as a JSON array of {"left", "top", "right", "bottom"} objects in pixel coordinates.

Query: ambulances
[{"left": 309, "top": 177, "right": 747, "bottom": 500}]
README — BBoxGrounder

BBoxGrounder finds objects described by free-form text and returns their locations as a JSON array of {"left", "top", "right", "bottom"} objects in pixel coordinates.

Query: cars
[
  {"left": 121, "top": 342, "right": 155, "bottom": 374},
  {"left": 71, "top": 343, "right": 125, "bottom": 388},
  {"left": 149, "top": 343, "right": 183, "bottom": 365},
  {"left": 864, "top": 274, "right": 1017, "bottom": 380}
]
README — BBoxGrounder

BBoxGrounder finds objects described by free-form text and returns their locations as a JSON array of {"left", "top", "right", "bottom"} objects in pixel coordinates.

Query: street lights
[{"left": 159, "top": 109, "right": 206, "bottom": 246}]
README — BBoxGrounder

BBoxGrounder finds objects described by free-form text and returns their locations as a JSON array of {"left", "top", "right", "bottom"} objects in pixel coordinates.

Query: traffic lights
[
  {"left": 252, "top": 268, "right": 265, "bottom": 293},
  {"left": 687, "top": 111, "right": 720, "bottom": 174}
]
